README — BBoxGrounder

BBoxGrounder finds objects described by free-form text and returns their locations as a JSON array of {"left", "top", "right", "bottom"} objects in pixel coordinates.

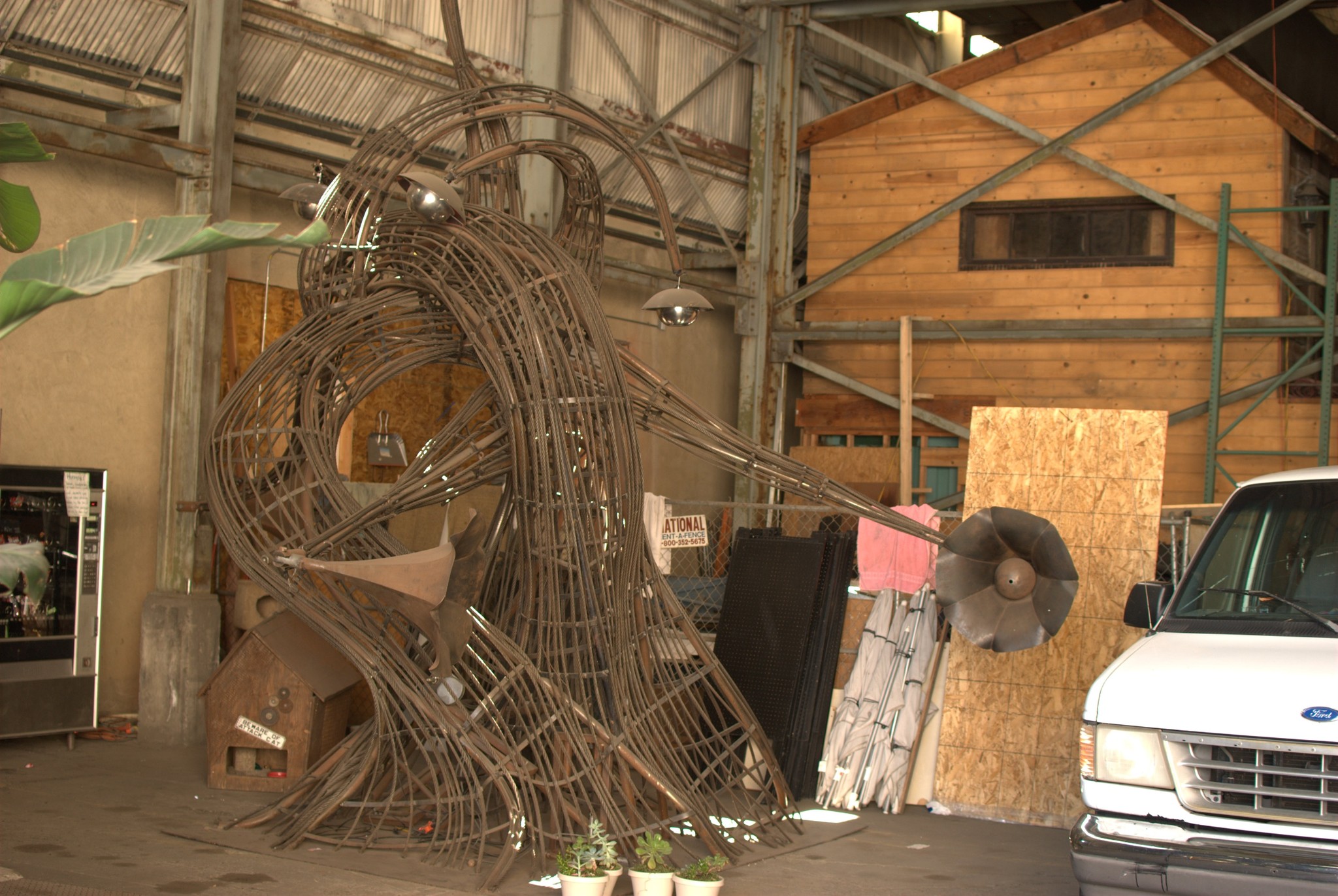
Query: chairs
[{"left": 1287, "top": 530, "right": 1338, "bottom": 625}]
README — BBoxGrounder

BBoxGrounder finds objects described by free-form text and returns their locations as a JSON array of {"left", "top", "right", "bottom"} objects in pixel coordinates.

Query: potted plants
[
  {"left": 586, "top": 818, "right": 623, "bottom": 896},
  {"left": 672, "top": 853, "right": 729, "bottom": 896},
  {"left": 627, "top": 831, "right": 676, "bottom": 896},
  {"left": 553, "top": 816, "right": 609, "bottom": 896}
]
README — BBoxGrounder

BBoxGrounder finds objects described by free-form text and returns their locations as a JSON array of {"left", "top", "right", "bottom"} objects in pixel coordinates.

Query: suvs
[{"left": 1064, "top": 466, "right": 1338, "bottom": 896}]
